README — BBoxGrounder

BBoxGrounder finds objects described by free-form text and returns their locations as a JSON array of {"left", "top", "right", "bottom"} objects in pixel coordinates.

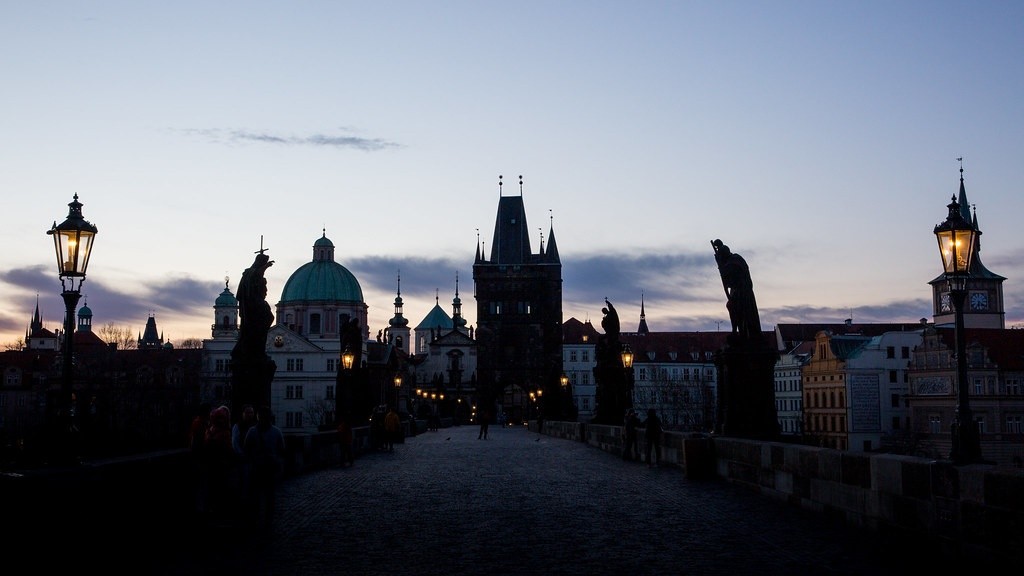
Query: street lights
[
  {"left": 618, "top": 342, "right": 636, "bottom": 426},
  {"left": 392, "top": 371, "right": 403, "bottom": 446},
  {"left": 44, "top": 193, "right": 98, "bottom": 474},
  {"left": 932, "top": 192, "right": 988, "bottom": 462},
  {"left": 339, "top": 343, "right": 357, "bottom": 467}
]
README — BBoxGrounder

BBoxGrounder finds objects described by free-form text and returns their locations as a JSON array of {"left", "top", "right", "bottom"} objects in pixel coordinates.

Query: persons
[
  {"left": 536, "top": 409, "right": 544, "bottom": 434},
  {"left": 477, "top": 412, "right": 492, "bottom": 442},
  {"left": 336, "top": 412, "right": 354, "bottom": 466},
  {"left": 602, "top": 300, "right": 620, "bottom": 336},
  {"left": 621, "top": 407, "right": 666, "bottom": 465},
  {"left": 715, "top": 246, "right": 760, "bottom": 334},
  {"left": 499, "top": 412, "right": 507, "bottom": 429},
  {"left": 371, "top": 406, "right": 401, "bottom": 451},
  {"left": 187, "top": 400, "right": 287, "bottom": 514},
  {"left": 235, "top": 253, "right": 275, "bottom": 360},
  {"left": 338, "top": 316, "right": 363, "bottom": 378}
]
